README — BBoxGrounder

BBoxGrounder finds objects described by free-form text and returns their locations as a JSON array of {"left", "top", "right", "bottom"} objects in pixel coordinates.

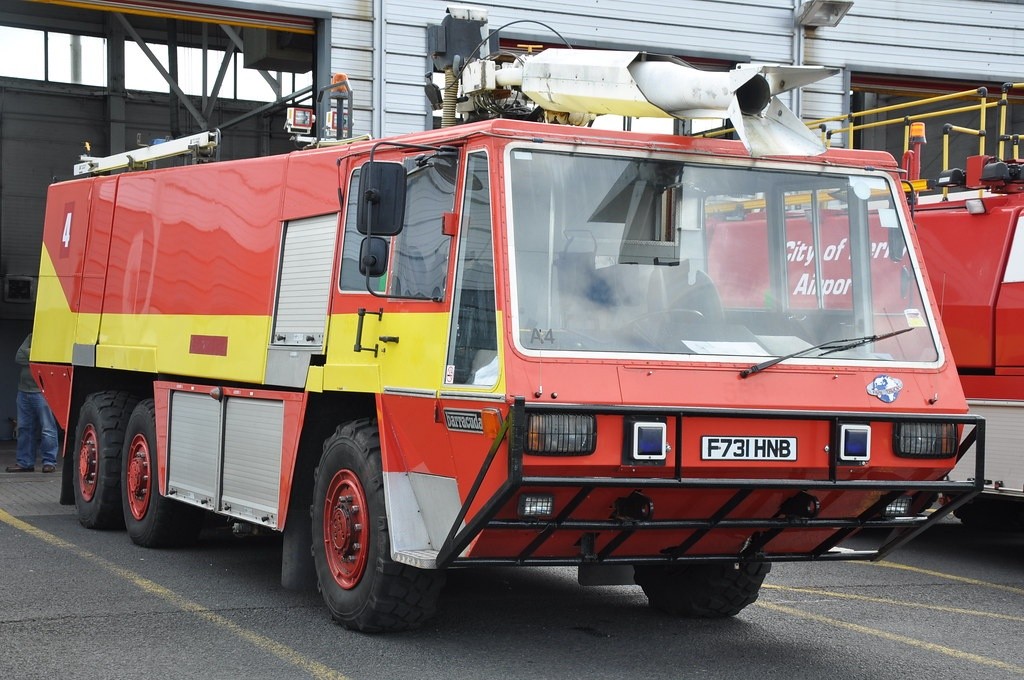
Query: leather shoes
[
  {"left": 6, "top": 464, "right": 35, "bottom": 472},
  {"left": 42, "top": 465, "right": 56, "bottom": 472}
]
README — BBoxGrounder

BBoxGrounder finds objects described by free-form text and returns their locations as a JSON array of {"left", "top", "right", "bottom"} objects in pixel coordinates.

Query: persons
[{"left": 5, "top": 333, "right": 58, "bottom": 473}]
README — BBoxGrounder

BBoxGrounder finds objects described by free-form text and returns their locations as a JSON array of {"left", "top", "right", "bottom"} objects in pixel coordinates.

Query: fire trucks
[
  {"left": 699, "top": 83, "right": 1024, "bottom": 516},
  {"left": 27, "top": 17, "right": 988, "bottom": 637}
]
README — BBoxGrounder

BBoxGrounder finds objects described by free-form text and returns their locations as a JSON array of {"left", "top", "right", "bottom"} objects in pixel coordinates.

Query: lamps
[{"left": 796, "top": 1, "right": 853, "bottom": 28}]
